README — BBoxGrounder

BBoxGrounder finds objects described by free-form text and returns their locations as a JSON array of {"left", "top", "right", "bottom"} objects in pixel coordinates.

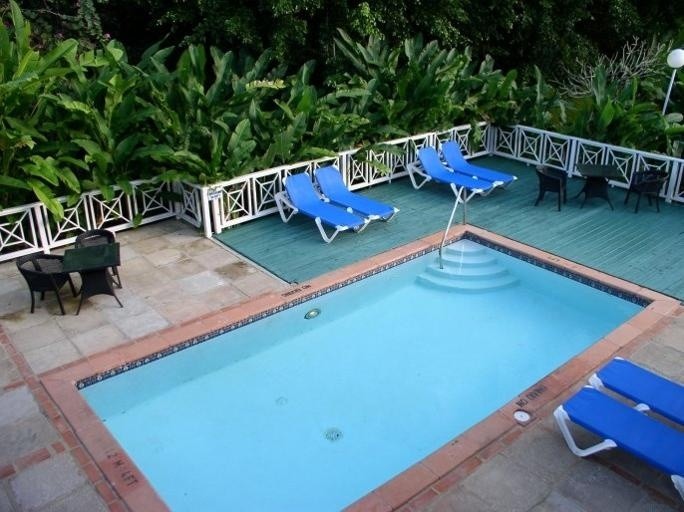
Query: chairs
[
  {"left": 535, "top": 163, "right": 567, "bottom": 211},
  {"left": 437, "top": 143, "right": 518, "bottom": 191},
  {"left": 589, "top": 356, "right": 682, "bottom": 425},
  {"left": 625, "top": 170, "right": 669, "bottom": 214},
  {"left": 407, "top": 147, "right": 498, "bottom": 205},
  {"left": 555, "top": 385, "right": 683, "bottom": 497},
  {"left": 313, "top": 166, "right": 399, "bottom": 223},
  {"left": 15, "top": 229, "right": 124, "bottom": 316},
  {"left": 275, "top": 172, "right": 370, "bottom": 242}
]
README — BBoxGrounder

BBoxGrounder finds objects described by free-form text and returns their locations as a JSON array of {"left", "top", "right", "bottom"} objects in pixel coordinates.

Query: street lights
[{"left": 661, "top": 46, "right": 684, "bottom": 117}]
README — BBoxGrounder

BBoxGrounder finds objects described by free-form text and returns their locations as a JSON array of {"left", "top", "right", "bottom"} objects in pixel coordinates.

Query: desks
[{"left": 572, "top": 164, "right": 619, "bottom": 211}]
